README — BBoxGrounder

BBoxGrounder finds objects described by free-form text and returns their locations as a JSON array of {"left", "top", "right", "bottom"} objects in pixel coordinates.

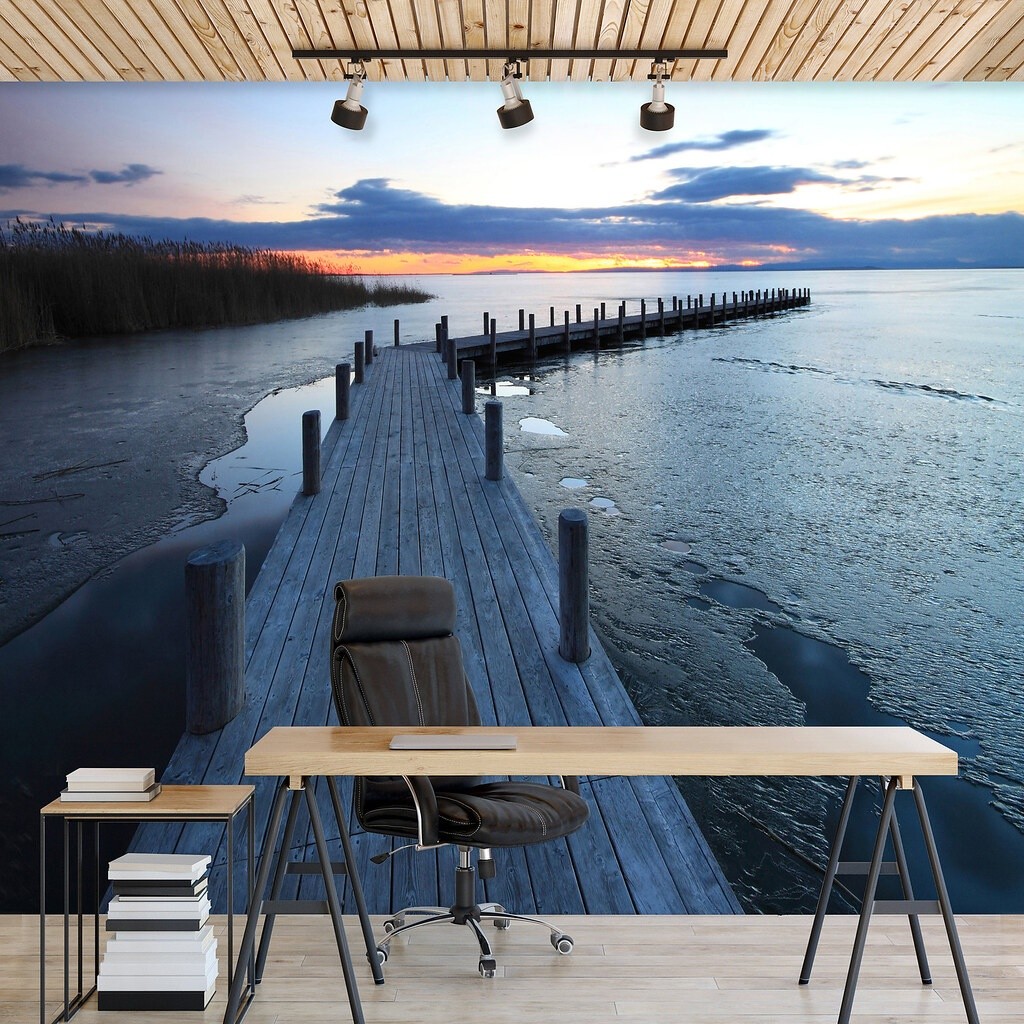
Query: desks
[
  {"left": 40, "top": 784, "right": 255, "bottom": 1024},
  {"left": 222, "top": 727, "right": 980, "bottom": 1024}
]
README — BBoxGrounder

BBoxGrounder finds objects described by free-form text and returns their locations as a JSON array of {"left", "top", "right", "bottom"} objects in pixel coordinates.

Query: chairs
[{"left": 331, "top": 576, "right": 590, "bottom": 979}]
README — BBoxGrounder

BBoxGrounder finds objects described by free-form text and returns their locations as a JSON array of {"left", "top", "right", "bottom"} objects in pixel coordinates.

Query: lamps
[
  {"left": 331, "top": 57, "right": 368, "bottom": 130},
  {"left": 496, "top": 57, "right": 534, "bottom": 129},
  {"left": 640, "top": 54, "right": 675, "bottom": 131}
]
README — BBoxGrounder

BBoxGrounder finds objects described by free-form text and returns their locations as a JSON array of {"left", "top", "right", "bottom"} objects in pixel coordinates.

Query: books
[
  {"left": 97, "top": 853, "right": 219, "bottom": 1011},
  {"left": 60, "top": 768, "right": 162, "bottom": 802}
]
